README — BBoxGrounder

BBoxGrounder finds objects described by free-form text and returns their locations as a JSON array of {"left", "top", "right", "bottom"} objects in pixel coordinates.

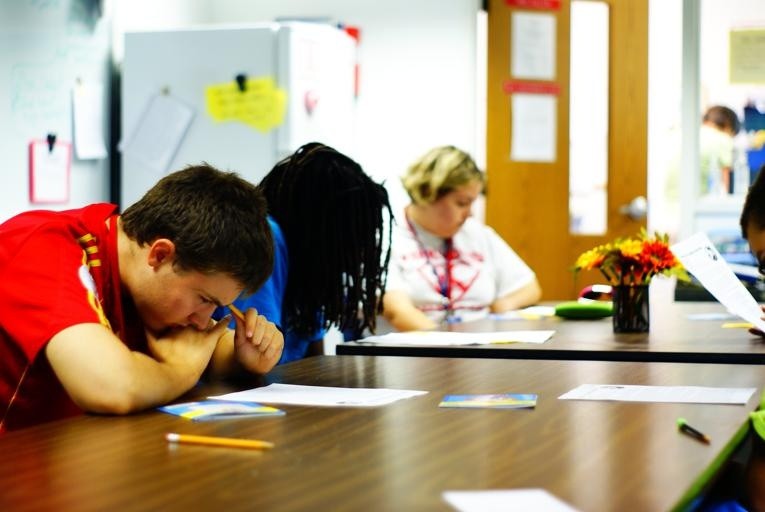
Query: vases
[{"left": 613, "top": 286, "right": 651, "bottom": 333}]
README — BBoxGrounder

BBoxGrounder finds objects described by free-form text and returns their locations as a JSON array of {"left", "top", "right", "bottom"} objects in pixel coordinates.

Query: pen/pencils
[
  {"left": 675, "top": 418, "right": 711, "bottom": 445},
  {"left": 228, "top": 303, "right": 246, "bottom": 323},
  {"left": 722, "top": 322, "right": 754, "bottom": 330},
  {"left": 164, "top": 432, "right": 275, "bottom": 448}
]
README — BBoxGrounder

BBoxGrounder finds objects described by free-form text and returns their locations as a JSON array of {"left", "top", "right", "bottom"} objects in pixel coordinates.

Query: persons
[
  {"left": 739, "top": 161, "right": 765, "bottom": 512},
  {"left": 1, "top": 163, "right": 284, "bottom": 437},
  {"left": 211, "top": 142, "right": 395, "bottom": 368},
  {"left": 357, "top": 145, "right": 542, "bottom": 338},
  {"left": 697, "top": 106, "right": 740, "bottom": 199}
]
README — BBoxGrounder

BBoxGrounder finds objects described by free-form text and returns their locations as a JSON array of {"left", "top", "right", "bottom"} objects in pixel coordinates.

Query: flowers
[{"left": 568, "top": 226, "right": 692, "bottom": 334}]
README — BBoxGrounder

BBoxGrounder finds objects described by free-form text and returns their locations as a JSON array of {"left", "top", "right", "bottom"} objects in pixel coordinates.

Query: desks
[
  {"left": 0, "top": 353, "right": 765, "bottom": 512},
  {"left": 335, "top": 299, "right": 765, "bottom": 364}
]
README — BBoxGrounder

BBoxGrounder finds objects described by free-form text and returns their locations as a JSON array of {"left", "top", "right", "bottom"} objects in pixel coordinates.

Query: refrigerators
[{"left": 121, "top": 23, "right": 364, "bottom": 225}]
